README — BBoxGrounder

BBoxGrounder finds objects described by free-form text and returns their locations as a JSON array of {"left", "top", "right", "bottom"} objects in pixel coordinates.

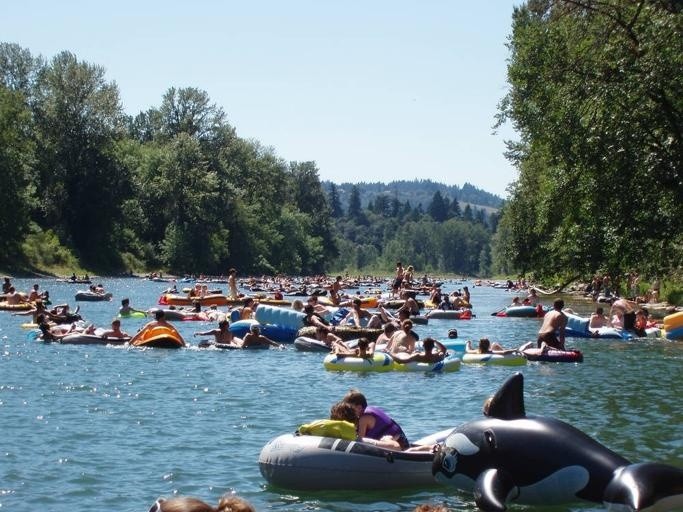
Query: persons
[
  {"left": 327, "top": 401, "right": 399, "bottom": 452},
  {"left": 342, "top": 387, "right": 408, "bottom": 447},
  {"left": 0, "top": 261, "right": 659, "bottom": 361}
]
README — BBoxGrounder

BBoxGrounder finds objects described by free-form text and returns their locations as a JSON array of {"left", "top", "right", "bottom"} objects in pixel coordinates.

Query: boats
[{"left": 258, "top": 424, "right": 460, "bottom": 489}]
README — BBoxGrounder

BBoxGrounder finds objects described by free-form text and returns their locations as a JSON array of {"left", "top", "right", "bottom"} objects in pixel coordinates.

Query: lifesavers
[
  {"left": 381, "top": 297, "right": 425, "bottom": 310},
  {"left": 183, "top": 287, "right": 222, "bottom": 294},
  {"left": 298, "top": 325, "right": 385, "bottom": 343},
  {"left": 662, "top": 311, "right": 683, "bottom": 339},
  {"left": 359, "top": 276, "right": 484, "bottom": 301},
  {"left": 132, "top": 324, "right": 183, "bottom": 348},
  {"left": 387, "top": 308, "right": 428, "bottom": 325},
  {"left": 227, "top": 272, "right": 337, "bottom": 305},
  {"left": 464, "top": 345, "right": 525, "bottom": 366},
  {"left": 372, "top": 339, "right": 426, "bottom": 354},
  {"left": 117, "top": 309, "right": 147, "bottom": 321},
  {"left": 316, "top": 294, "right": 378, "bottom": 308},
  {"left": 394, "top": 353, "right": 461, "bottom": 372},
  {"left": 431, "top": 370, "right": 681, "bottom": 511},
  {"left": 254, "top": 305, "right": 332, "bottom": 329},
  {"left": 163, "top": 288, "right": 180, "bottom": 294},
  {"left": 427, "top": 307, "right": 470, "bottom": 318},
  {"left": 523, "top": 348, "right": 584, "bottom": 363},
  {"left": 491, "top": 306, "right": 554, "bottom": 317},
  {"left": 435, "top": 336, "right": 466, "bottom": 352},
  {"left": 324, "top": 351, "right": 394, "bottom": 373},
  {"left": 199, "top": 338, "right": 269, "bottom": 350},
  {"left": 159, "top": 293, "right": 227, "bottom": 306},
  {"left": 331, "top": 308, "right": 373, "bottom": 329},
  {"left": 424, "top": 296, "right": 470, "bottom": 309},
  {"left": 150, "top": 306, "right": 214, "bottom": 321},
  {"left": 228, "top": 310, "right": 301, "bottom": 343},
  {"left": 199, "top": 309, "right": 227, "bottom": 322},
  {"left": 294, "top": 335, "right": 368, "bottom": 351}
]
